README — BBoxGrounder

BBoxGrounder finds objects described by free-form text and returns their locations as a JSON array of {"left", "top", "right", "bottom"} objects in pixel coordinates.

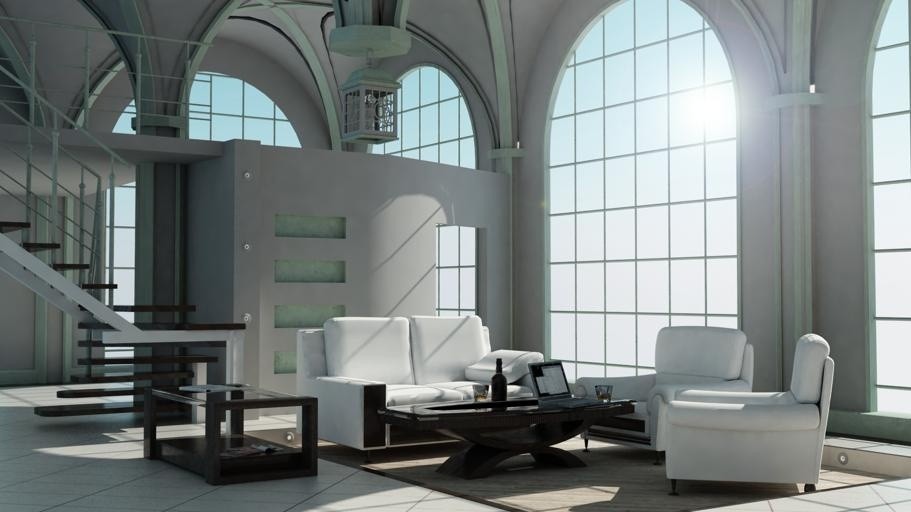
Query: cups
[
  {"left": 473, "top": 384, "right": 490, "bottom": 401},
  {"left": 594, "top": 384, "right": 614, "bottom": 402},
  {"left": 570, "top": 381, "right": 589, "bottom": 399}
]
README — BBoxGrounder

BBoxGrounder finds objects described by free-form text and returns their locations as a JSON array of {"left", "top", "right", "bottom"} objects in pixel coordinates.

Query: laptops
[{"left": 529, "top": 361, "right": 602, "bottom": 413}]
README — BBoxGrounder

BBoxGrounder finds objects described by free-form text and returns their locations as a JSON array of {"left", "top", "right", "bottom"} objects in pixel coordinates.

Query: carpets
[{"left": 361, "top": 434, "right": 896, "bottom": 512}]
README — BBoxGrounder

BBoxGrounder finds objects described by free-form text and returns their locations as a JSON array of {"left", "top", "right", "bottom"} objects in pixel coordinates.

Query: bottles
[{"left": 491, "top": 357, "right": 508, "bottom": 411}]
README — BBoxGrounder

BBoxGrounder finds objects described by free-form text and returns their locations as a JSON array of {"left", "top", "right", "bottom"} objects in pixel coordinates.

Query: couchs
[
  {"left": 665, "top": 333, "right": 834, "bottom": 496},
  {"left": 296, "top": 315, "right": 544, "bottom": 464},
  {"left": 574, "top": 326, "right": 754, "bottom": 466}
]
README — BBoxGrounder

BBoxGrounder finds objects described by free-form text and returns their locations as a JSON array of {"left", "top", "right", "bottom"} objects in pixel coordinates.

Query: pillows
[{"left": 465, "top": 349, "right": 544, "bottom": 384}]
